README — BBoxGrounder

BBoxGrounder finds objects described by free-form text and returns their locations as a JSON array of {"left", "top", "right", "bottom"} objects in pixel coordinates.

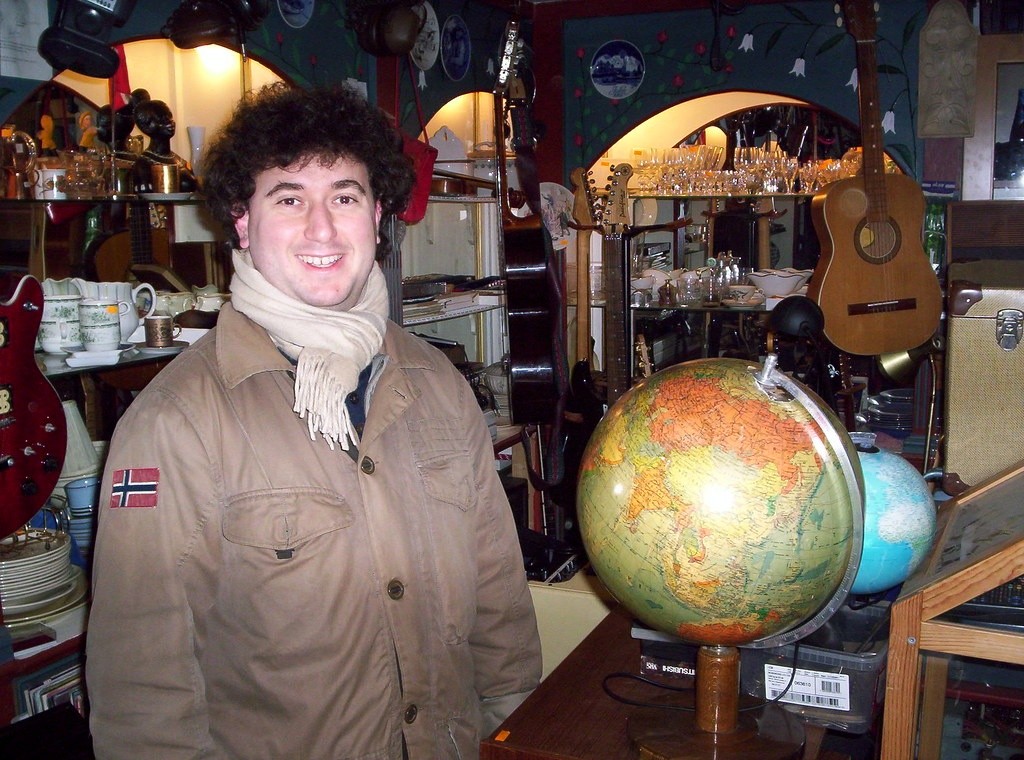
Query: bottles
[
  {"left": 656, "top": 277, "right": 677, "bottom": 306},
  {"left": 75, "top": 204, "right": 105, "bottom": 284}
]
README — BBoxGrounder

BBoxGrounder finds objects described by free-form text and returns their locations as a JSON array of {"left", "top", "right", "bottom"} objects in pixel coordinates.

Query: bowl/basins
[
  {"left": 43, "top": 439, "right": 110, "bottom": 509},
  {"left": 746, "top": 264, "right": 815, "bottom": 298},
  {"left": 56, "top": 506, "right": 99, "bottom": 556}
]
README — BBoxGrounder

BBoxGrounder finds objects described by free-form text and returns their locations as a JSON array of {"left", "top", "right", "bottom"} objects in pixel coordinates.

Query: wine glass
[{"left": 631, "top": 143, "right": 842, "bottom": 196}]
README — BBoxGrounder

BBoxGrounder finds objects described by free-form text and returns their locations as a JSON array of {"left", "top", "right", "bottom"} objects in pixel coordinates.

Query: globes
[
  {"left": 847, "top": 430, "right": 937, "bottom": 597},
  {"left": 577, "top": 353, "right": 866, "bottom": 760}
]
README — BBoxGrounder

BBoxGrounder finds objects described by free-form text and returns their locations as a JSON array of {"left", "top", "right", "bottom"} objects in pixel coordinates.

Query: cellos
[{"left": 492, "top": 18, "right": 564, "bottom": 539}]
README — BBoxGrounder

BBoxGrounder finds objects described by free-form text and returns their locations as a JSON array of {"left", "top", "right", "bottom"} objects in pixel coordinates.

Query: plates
[
  {"left": 720, "top": 296, "right": 764, "bottom": 311},
  {"left": 60, "top": 342, "right": 137, "bottom": 354},
  {"left": 0, "top": 527, "right": 90, "bottom": 631},
  {"left": 135, "top": 340, "right": 189, "bottom": 355}
]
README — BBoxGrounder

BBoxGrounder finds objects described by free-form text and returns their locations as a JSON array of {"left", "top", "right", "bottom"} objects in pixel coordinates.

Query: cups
[
  {"left": 696, "top": 275, "right": 718, "bottom": 306},
  {"left": 152, "top": 283, "right": 195, "bottom": 321},
  {"left": 34, "top": 167, "right": 68, "bottom": 201},
  {"left": 59, "top": 399, "right": 101, "bottom": 479},
  {"left": 77, "top": 298, "right": 130, "bottom": 350},
  {"left": 38, "top": 294, "right": 82, "bottom": 355},
  {"left": 151, "top": 163, "right": 180, "bottom": 195},
  {"left": 63, "top": 475, "right": 100, "bottom": 519},
  {"left": 628, "top": 263, "right": 711, "bottom": 305},
  {"left": 675, "top": 274, "right": 701, "bottom": 309},
  {"left": 144, "top": 315, "right": 181, "bottom": 347},
  {"left": 728, "top": 285, "right": 764, "bottom": 302}
]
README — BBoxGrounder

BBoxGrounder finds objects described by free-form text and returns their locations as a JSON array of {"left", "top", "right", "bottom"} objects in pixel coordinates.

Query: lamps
[{"left": 770, "top": 294, "right": 841, "bottom": 421}]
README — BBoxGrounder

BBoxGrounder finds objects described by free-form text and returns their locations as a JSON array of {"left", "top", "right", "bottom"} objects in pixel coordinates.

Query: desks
[{"left": 478, "top": 607, "right": 865, "bottom": 759}]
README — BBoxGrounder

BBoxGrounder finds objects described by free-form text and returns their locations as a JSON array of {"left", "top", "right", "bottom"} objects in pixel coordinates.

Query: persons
[
  {"left": 86, "top": 81, "right": 542, "bottom": 760},
  {"left": 126, "top": 100, "right": 199, "bottom": 193}
]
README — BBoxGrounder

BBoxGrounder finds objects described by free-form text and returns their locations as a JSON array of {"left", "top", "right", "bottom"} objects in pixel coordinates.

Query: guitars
[
  {"left": 569, "top": 161, "right": 656, "bottom": 471},
  {"left": 1, "top": 275, "right": 69, "bottom": 544},
  {"left": 807, "top": 0, "right": 942, "bottom": 357}
]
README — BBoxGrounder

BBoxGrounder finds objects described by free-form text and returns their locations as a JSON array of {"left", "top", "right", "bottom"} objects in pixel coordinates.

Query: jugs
[
  {"left": 0, "top": 123, "right": 36, "bottom": 201},
  {"left": 38, "top": 277, "right": 157, "bottom": 347},
  {"left": 189, "top": 284, "right": 232, "bottom": 319},
  {"left": 55, "top": 147, "right": 108, "bottom": 200},
  {"left": 695, "top": 249, "right": 744, "bottom": 310}
]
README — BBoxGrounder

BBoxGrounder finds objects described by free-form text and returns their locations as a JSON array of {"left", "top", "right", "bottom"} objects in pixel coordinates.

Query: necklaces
[{"left": 144, "top": 149, "right": 176, "bottom": 160}]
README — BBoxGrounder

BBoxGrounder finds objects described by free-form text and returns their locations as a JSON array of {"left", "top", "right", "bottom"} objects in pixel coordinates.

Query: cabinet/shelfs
[
  {"left": 0, "top": 194, "right": 505, "bottom": 760},
  {"left": 623, "top": 194, "right": 811, "bottom": 382}
]
